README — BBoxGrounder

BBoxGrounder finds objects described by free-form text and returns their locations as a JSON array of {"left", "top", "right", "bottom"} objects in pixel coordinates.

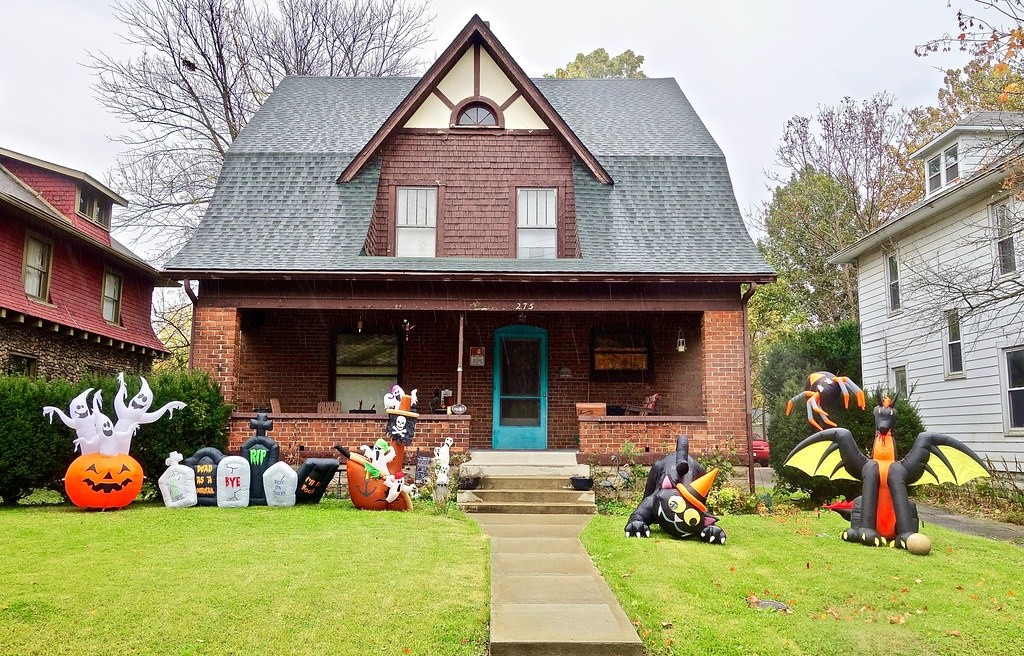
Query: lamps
[{"left": 675, "top": 328, "right": 687, "bottom": 352}]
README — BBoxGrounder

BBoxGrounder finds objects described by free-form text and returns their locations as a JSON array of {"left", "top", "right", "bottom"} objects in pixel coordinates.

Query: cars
[{"left": 752, "top": 432, "right": 770, "bottom": 466}]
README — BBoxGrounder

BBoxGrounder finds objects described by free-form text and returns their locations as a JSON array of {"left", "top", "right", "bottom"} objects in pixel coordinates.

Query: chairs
[
  {"left": 317, "top": 401, "right": 342, "bottom": 413},
  {"left": 271, "top": 398, "right": 281, "bottom": 413}
]
